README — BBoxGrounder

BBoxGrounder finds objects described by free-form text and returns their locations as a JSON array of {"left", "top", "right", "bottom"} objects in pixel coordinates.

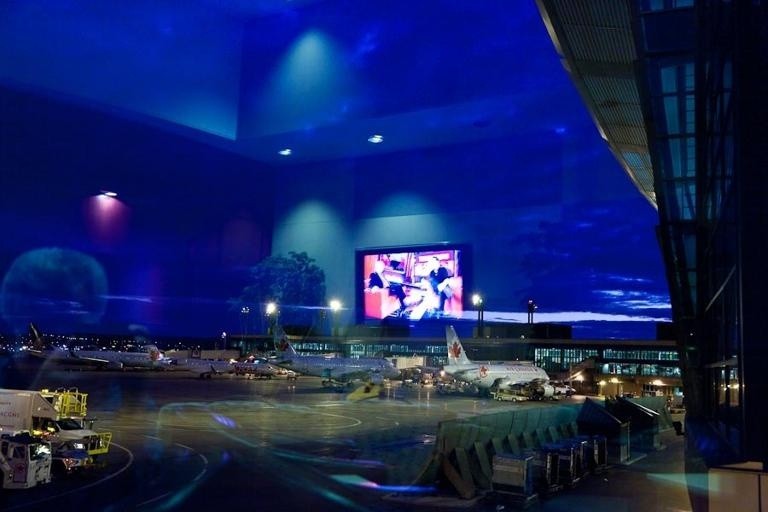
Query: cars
[{"left": 555, "top": 385, "right": 577, "bottom": 395}]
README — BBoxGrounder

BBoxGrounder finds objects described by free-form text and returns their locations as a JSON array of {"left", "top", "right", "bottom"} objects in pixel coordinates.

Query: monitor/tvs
[{"left": 354, "top": 241, "right": 471, "bottom": 324}]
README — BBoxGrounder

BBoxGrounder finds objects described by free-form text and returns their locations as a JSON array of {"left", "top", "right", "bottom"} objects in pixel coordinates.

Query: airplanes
[
  {"left": 143, "top": 345, "right": 275, "bottom": 379},
  {"left": 28, "top": 323, "right": 172, "bottom": 370},
  {"left": 266, "top": 326, "right": 402, "bottom": 393},
  {"left": 443, "top": 326, "right": 554, "bottom": 401}
]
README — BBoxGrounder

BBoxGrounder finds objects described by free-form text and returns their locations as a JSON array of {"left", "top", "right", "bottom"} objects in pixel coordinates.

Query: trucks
[{"left": 0, "top": 388, "right": 113, "bottom": 491}]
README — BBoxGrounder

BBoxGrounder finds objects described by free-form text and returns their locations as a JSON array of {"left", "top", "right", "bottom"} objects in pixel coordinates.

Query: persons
[
  {"left": 429, "top": 256, "right": 452, "bottom": 316},
  {"left": 370, "top": 259, "right": 409, "bottom": 309}
]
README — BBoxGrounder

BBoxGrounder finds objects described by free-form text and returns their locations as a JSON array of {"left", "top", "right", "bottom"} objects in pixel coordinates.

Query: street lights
[
  {"left": 265, "top": 302, "right": 280, "bottom": 336},
  {"left": 471, "top": 293, "right": 484, "bottom": 339},
  {"left": 241, "top": 307, "right": 250, "bottom": 354},
  {"left": 328, "top": 299, "right": 343, "bottom": 336}
]
